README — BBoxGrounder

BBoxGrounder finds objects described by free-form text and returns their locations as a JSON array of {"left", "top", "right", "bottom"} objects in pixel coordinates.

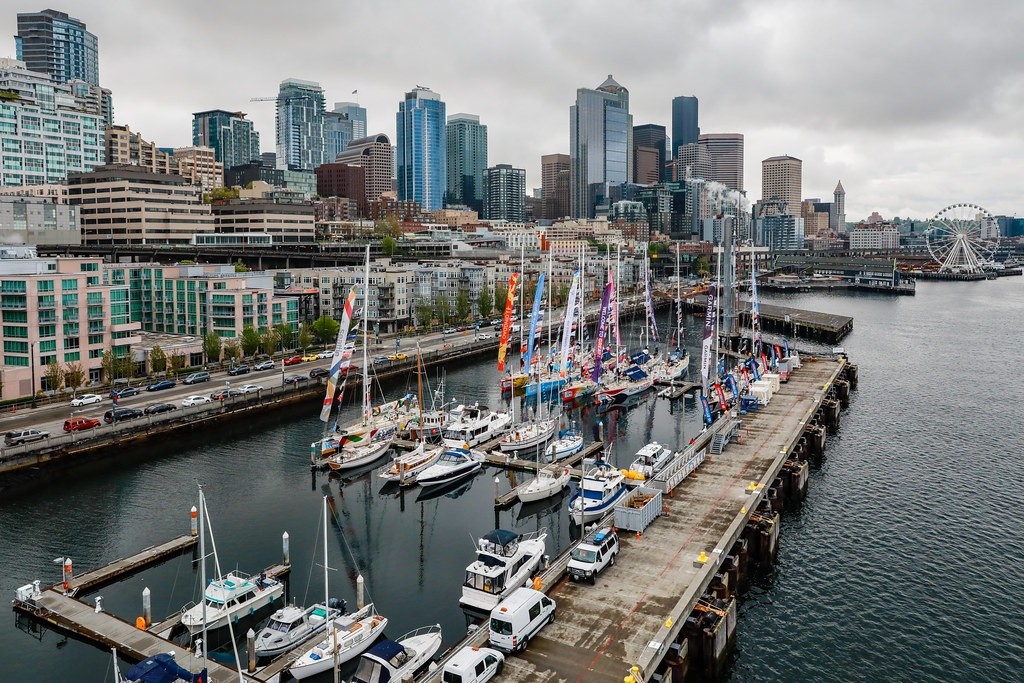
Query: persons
[
  {"left": 745, "top": 352, "right": 755, "bottom": 374},
  {"left": 622, "top": 353, "right": 627, "bottom": 363},
  {"left": 676, "top": 348, "right": 680, "bottom": 352},
  {"left": 606, "top": 344, "right": 610, "bottom": 352}
]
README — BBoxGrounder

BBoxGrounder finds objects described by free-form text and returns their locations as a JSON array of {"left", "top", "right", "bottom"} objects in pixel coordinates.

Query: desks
[
  {"left": 749, "top": 386, "right": 770, "bottom": 407},
  {"left": 752, "top": 380, "right": 773, "bottom": 400},
  {"left": 761, "top": 374, "right": 780, "bottom": 394}
]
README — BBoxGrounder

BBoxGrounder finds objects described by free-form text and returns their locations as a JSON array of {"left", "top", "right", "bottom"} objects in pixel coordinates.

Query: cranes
[{"left": 250, "top": 94, "right": 312, "bottom": 164}]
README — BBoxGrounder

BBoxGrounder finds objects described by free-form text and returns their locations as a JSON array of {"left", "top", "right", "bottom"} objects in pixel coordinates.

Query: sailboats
[
  {"left": 500, "top": 233, "right": 690, "bottom": 404},
  {"left": 309, "top": 242, "right": 465, "bottom": 484},
  {"left": 181, "top": 484, "right": 284, "bottom": 634},
  {"left": 498, "top": 345, "right": 586, "bottom": 502},
  {"left": 703, "top": 236, "right": 777, "bottom": 415},
  {"left": 286, "top": 493, "right": 390, "bottom": 679}
]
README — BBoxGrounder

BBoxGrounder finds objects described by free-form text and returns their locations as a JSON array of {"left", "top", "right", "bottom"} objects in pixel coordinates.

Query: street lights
[{"left": 31, "top": 340, "right": 38, "bottom": 406}]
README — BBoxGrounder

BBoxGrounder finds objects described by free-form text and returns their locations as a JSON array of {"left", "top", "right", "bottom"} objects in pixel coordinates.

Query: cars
[
  {"left": 144, "top": 385, "right": 264, "bottom": 413},
  {"left": 442, "top": 313, "right": 534, "bottom": 342},
  {"left": 70, "top": 376, "right": 178, "bottom": 407},
  {"left": 226, "top": 344, "right": 357, "bottom": 376},
  {"left": 285, "top": 354, "right": 409, "bottom": 384}
]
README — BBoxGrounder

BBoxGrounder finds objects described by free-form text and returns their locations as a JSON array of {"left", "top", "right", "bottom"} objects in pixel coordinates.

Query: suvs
[
  {"left": 62, "top": 406, "right": 144, "bottom": 433},
  {"left": 183, "top": 371, "right": 212, "bottom": 385},
  {"left": 4, "top": 428, "right": 52, "bottom": 447}
]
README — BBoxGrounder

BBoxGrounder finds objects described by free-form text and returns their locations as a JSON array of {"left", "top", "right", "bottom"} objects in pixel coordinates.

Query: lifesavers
[{"left": 533, "top": 576, "right": 543, "bottom": 590}]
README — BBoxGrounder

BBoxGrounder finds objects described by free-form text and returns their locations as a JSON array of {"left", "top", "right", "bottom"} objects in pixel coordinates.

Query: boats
[
  {"left": 458, "top": 523, "right": 551, "bottom": 621},
  {"left": 417, "top": 400, "right": 512, "bottom": 486},
  {"left": 245, "top": 597, "right": 347, "bottom": 656},
  {"left": 347, "top": 622, "right": 443, "bottom": 683},
  {"left": 571, "top": 439, "right": 676, "bottom": 527}
]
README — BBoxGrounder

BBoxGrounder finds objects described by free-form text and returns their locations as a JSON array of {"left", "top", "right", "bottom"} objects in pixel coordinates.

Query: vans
[
  {"left": 564, "top": 527, "right": 620, "bottom": 586},
  {"left": 439, "top": 584, "right": 558, "bottom": 683}
]
